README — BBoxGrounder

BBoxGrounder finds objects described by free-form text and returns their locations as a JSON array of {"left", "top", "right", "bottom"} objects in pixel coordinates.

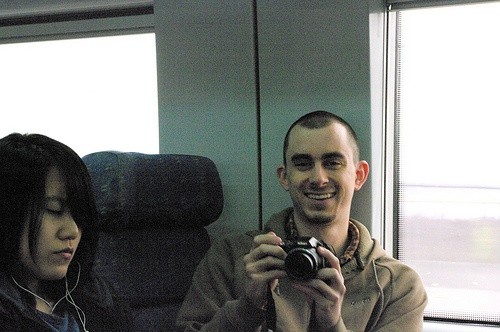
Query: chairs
[{"left": 81, "top": 152, "right": 226, "bottom": 332}]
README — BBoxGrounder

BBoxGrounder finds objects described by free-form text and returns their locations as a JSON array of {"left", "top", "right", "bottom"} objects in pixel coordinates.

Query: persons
[
  {"left": 0, "top": 131, "right": 104, "bottom": 331},
  {"left": 175, "top": 110, "right": 429, "bottom": 331}
]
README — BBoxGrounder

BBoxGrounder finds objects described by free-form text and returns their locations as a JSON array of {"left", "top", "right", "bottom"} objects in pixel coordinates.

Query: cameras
[{"left": 277, "top": 237, "right": 335, "bottom": 280}]
